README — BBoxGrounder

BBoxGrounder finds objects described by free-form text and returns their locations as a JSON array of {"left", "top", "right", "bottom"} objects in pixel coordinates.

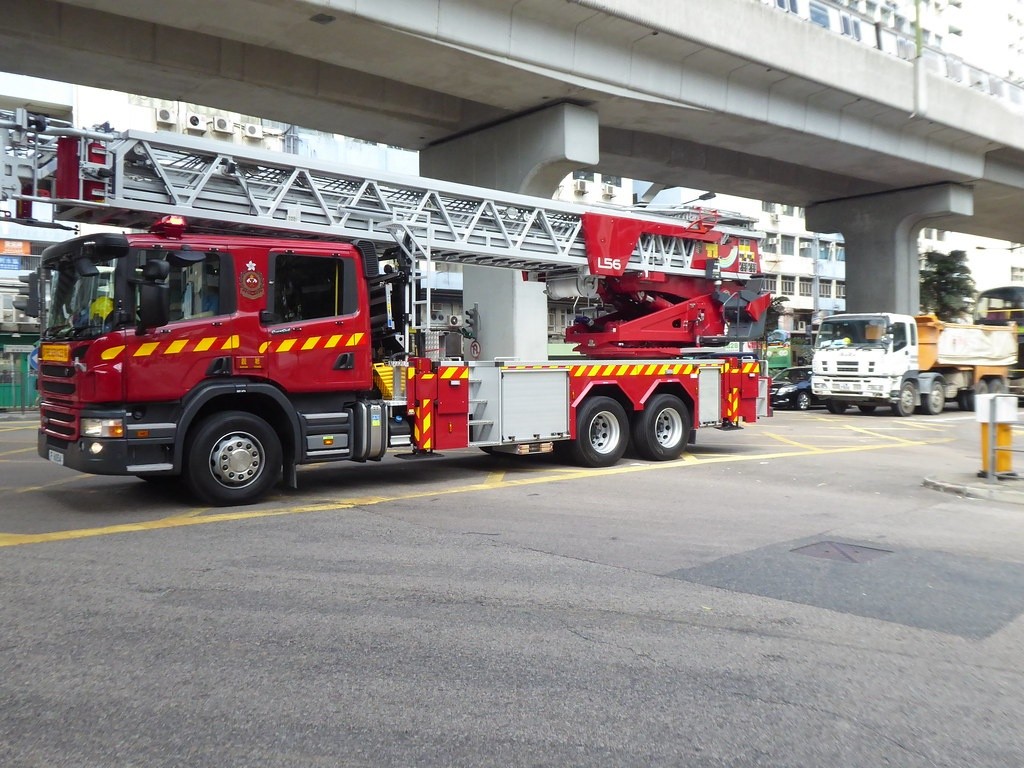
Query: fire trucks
[{"left": 0, "top": 106, "right": 775, "bottom": 510}]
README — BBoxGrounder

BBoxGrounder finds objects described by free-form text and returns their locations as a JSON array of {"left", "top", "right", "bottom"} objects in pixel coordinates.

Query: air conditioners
[
  {"left": 432, "top": 302, "right": 443, "bottom": 313},
  {"left": 574, "top": 179, "right": 587, "bottom": 194},
  {"left": 603, "top": 184, "right": 614, "bottom": 195},
  {"left": 769, "top": 238, "right": 779, "bottom": 245},
  {"left": 156, "top": 106, "right": 177, "bottom": 126},
  {"left": 801, "top": 242, "right": 811, "bottom": 249},
  {"left": 182, "top": 110, "right": 207, "bottom": 132},
  {"left": 448, "top": 314, "right": 464, "bottom": 326},
  {"left": 774, "top": 213, "right": 780, "bottom": 223},
  {"left": 245, "top": 123, "right": 264, "bottom": 139},
  {"left": 213, "top": 116, "right": 234, "bottom": 134}
]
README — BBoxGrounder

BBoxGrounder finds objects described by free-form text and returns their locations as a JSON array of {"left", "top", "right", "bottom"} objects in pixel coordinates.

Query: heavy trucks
[{"left": 805, "top": 311, "right": 1018, "bottom": 418}]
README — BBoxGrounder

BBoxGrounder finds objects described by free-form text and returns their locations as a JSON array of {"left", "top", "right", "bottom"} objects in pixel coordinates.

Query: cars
[{"left": 769, "top": 365, "right": 852, "bottom": 411}]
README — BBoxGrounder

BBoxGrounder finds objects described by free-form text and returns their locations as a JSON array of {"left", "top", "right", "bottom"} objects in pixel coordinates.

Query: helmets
[
  {"left": 843, "top": 337, "right": 851, "bottom": 345},
  {"left": 88, "top": 296, "right": 115, "bottom": 320}
]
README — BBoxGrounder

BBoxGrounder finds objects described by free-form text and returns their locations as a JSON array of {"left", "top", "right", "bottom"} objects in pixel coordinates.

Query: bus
[
  {"left": 972, "top": 285, "right": 1024, "bottom": 379},
  {"left": 767, "top": 329, "right": 817, "bottom": 372}
]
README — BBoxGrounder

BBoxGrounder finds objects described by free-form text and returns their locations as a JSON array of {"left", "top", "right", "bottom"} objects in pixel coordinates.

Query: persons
[{"left": 168, "top": 264, "right": 220, "bottom": 325}]
